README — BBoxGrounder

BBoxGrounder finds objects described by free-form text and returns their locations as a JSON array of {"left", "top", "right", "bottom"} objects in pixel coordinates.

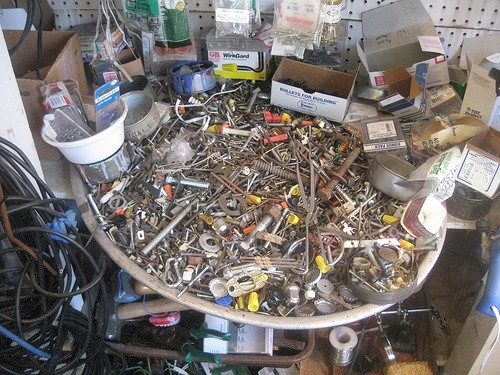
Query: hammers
[{"left": 105, "top": 295, "right": 196, "bottom": 341}]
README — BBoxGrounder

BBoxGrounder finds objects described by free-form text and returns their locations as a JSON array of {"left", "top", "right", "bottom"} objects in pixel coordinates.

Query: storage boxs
[
  {"left": 41, "top": 21, "right": 194, "bottom": 125},
  {"left": 3, "top": 30, "right": 88, "bottom": 159},
  {"left": 357, "top": 0, "right": 500, "bottom": 201},
  {"left": 271, "top": 55, "right": 361, "bottom": 123},
  {"left": 205, "top": 25, "right": 271, "bottom": 80}
]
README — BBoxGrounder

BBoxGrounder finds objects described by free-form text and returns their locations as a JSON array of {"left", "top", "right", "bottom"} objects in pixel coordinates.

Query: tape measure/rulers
[{"left": 149, "top": 311, "right": 181, "bottom": 326}]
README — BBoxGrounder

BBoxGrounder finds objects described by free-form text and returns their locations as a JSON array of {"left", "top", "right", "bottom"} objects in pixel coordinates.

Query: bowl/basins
[
  {"left": 170, "top": 60, "right": 216, "bottom": 94},
  {"left": 120, "top": 90, "right": 160, "bottom": 141},
  {"left": 120, "top": 75, "right": 156, "bottom": 100},
  {"left": 367, "top": 152, "right": 425, "bottom": 200},
  {"left": 41, "top": 98, "right": 128, "bottom": 164},
  {"left": 76, "top": 142, "right": 135, "bottom": 182},
  {"left": 446, "top": 181, "right": 491, "bottom": 220}
]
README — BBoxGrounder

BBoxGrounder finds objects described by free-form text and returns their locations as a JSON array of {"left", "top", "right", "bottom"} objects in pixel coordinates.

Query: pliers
[{"left": 136, "top": 321, "right": 233, "bottom": 365}]
